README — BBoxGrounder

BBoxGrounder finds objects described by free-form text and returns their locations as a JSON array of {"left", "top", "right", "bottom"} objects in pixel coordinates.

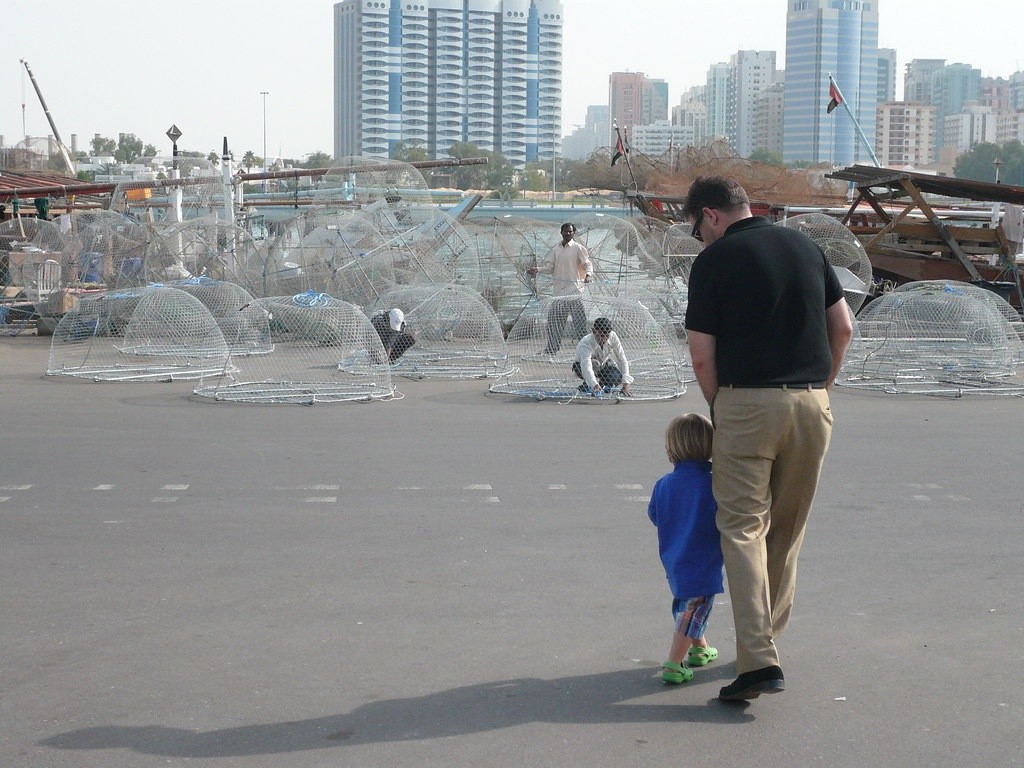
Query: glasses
[{"left": 691, "top": 207, "right": 713, "bottom": 243}]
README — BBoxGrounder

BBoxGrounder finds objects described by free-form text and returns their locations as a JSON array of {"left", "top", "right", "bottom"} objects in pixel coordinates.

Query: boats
[
  {"left": 0, "top": 193, "right": 484, "bottom": 333},
  {"left": 626, "top": 163, "right": 1024, "bottom": 316}
]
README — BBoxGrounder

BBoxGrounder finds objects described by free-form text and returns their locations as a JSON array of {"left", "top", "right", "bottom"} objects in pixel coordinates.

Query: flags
[
  {"left": 611, "top": 137, "right": 624, "bottom": 167},
  {"left": 826, "top": 82, "right": 841, "bottom": 114}
]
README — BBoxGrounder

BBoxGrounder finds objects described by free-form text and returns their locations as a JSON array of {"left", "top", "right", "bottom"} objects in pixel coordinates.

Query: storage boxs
[
  {"left": 48, "top": 290, "right": 80, "bottom": 315},
  {"left": 857, "top": 233, "right": 898, "bottom": 244}
]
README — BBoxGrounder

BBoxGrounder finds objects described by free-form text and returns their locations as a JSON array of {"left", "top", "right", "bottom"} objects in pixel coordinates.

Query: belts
[{"left": 719, "top": 381, "right": 827, "bottom": 388}]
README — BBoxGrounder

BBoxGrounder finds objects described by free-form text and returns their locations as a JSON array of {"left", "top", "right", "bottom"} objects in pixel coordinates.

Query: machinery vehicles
[{"left": 20, "top": 59, "right": 76, "bottom": 178}]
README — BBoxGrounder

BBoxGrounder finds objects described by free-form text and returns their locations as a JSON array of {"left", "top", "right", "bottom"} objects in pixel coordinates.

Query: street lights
[
  {"left": 260, "top": 92, "right": 271, "bottom": 193},
  {"left": 549, "top": 97, "right": 559, "bottom": 201}
]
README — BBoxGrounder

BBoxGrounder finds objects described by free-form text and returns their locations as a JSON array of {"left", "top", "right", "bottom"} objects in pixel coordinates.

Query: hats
[{"left": 389, "top": 308, "right": 404, "bottom": 331}]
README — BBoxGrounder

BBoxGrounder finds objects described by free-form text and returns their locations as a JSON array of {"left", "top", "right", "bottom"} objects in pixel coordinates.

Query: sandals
[
  {"left": 688, "top": 646, "right": 719, "bottom": 666},
  {"left": 661, "top": 661, "right": 694, "bottom": 683}
]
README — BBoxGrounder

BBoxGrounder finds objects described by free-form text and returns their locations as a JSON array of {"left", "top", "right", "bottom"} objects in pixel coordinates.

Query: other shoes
[{"left": 718, "top": 665, "right": 785, "bottom": 702}]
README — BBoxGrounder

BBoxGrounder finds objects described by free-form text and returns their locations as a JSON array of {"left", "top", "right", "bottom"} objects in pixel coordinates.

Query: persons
[
  {"left": 647, "top": 413, "right": 724, "bottom": 684},
  {"left": 572, "top": 317, "right": 634, "bottom": 397},
  {"left": 364, "top": 308, "right": 415, "bottom": 365},
  {"left": 683, "top": 174, "right": 853, "bottom": 699},
  {"left": 525, "top": 223, "right": 593, "bottom": 355}
]
